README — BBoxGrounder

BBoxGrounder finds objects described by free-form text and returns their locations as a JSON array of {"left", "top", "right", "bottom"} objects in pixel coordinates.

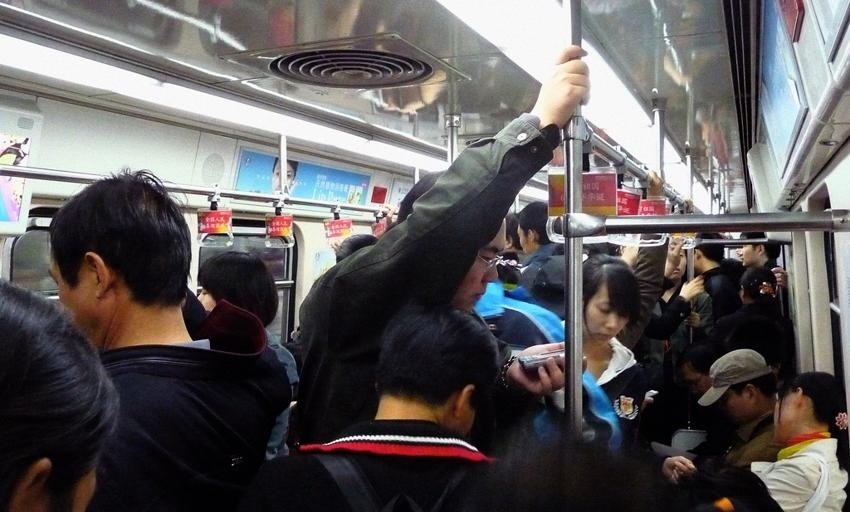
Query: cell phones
[{"left": 518, "top": 349, "right": 565, "bottom": 370}]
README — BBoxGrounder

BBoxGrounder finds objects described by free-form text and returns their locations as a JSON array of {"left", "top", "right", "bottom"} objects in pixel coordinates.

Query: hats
[{"left": 697, "top": 349, "right": 773, "bottom": 407}]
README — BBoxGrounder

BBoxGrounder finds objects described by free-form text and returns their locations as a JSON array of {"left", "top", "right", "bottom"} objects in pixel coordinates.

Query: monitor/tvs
[{"left": 0, "top": 91, "right": 45, "bottom": 237}]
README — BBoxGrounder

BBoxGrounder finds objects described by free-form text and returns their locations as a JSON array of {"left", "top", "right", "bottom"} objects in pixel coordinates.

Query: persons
[
  {"left": 0, "top": 278, "right": 118, "bottom": 512},
  {"left": 196, "top": 250, "right": 299, "bottom": 511},
  {"left": 294, "top": 306, "right": 499, "bottom": 510},
  {"left": 273, "top": 158, "right": 298, "bottom": 195},
  {"left": 331, "top": 207, "right": 395, "bottom": 261},
  {"left": 49, "top": 169, "right": 292, "bottom": 511},
  {"left": 477, "top": 201, "right": 849, "bottom": 512},
  {"left": 296, "top": 45, "right": 590, "bottom": 444}
]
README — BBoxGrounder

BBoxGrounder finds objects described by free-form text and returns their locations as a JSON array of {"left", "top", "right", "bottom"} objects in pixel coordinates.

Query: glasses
[{"left": 478, "top": 255, "right": 503, "bottom": 273}]
